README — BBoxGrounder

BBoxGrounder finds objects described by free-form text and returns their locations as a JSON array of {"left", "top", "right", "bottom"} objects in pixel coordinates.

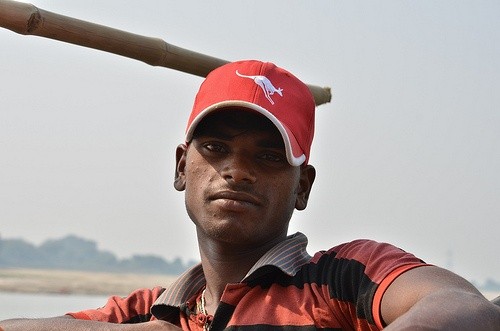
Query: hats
[{"left": 187, "top": 60, "right": 315, "bottom": 167}]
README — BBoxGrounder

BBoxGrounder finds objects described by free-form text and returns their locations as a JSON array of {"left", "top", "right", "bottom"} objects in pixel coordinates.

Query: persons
[{"left": 0, "top": 60, "right": 500, "bottom": 331}]
